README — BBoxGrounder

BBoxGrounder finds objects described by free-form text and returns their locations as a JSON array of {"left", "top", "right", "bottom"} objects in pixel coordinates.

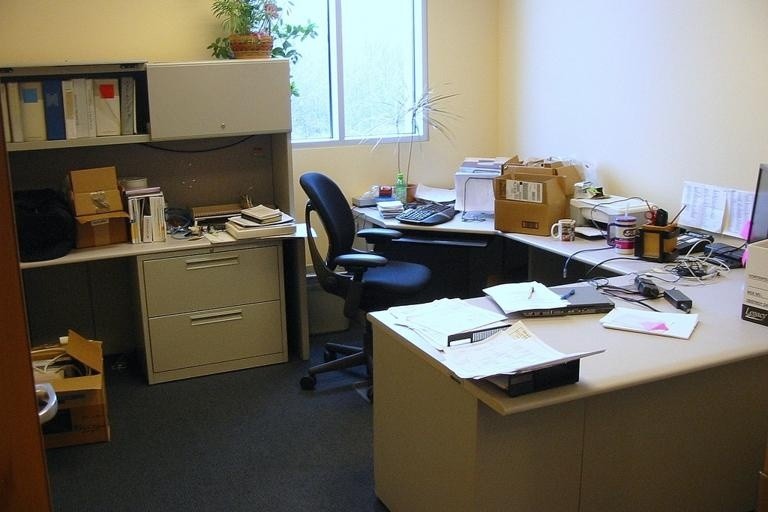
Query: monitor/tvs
[{"left": 747, "top": 164, "right": 768, "bottom": 244}]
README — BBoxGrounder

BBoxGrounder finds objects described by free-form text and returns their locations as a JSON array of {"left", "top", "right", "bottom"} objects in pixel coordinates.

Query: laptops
[{"left": 518, "top": 286, "right": 615, "bottom": 317}]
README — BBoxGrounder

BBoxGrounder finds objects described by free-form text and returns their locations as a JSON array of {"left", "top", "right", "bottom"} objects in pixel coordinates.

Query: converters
[
  {"left": 664, "top": 290, "right": 692, "bottom": 309},
  {"left": 634, "top": 278, "right": 659, "bottom": 296}
]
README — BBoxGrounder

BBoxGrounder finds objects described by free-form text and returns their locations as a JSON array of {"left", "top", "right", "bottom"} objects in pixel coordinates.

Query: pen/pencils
[
  {"left": 527, "top": 284, "right": 537, "bottom": 301},
  {"left": 560, "top": 288, "right": 578, "bottom": 300}
]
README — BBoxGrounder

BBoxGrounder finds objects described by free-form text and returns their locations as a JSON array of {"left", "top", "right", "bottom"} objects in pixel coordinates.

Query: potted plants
[
  {"left": 205, "top": 0, "right": 320, "bottom": 104},
  {"left": 360, "top": 80, "right": 464, "bottom": 203}
]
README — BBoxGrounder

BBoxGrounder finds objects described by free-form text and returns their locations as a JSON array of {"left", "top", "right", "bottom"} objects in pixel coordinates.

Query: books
[
  {"left": 224, "top": 204, "right": 298, "bottom": 240},
  {"left": 127, "top": 192, "right": 168, "bottom": 243}
]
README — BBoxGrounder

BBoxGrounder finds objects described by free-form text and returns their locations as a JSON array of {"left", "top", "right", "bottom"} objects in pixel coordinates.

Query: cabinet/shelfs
[
  {"left": 0, "top": 58, "right": 312, "bottom": 361},
  {"left": 128, "top": 238, "right": 290, "bottom": 386}
]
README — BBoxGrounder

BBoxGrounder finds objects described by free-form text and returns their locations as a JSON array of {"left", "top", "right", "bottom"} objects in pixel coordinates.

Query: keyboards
[{"left": 396, "top": 203, "right": 460, "bottom": 225}]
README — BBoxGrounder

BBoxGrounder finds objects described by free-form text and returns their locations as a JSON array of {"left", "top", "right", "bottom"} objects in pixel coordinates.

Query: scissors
[{"left": 644, "top": 205, "right": 659, "bottom": 226}]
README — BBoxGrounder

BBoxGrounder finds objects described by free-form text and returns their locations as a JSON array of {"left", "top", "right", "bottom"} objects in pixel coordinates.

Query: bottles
[{"left": 395, "top": 173, "right": 408, "bottom": 210}]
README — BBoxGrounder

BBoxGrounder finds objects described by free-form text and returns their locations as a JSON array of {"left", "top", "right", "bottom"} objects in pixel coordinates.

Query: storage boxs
[
  {"left": 69, "top": 165, "right": 120, "bottom": 196},
  {"left": 68, "top": 188, "right": 124, "bottom": 217},
  {"left": 30, "top": 327, "right": 113, "bottom": 448},
  {"left": 499, "top": 152, "right": 585, "bottom": 219},
  {"left": 77, "top": 211, "right": 130, "bottom": 252},
  {"left": 492, "top": 175, "right": 567, "bottom": 238},
  {"left": 739, "top": 238, "right": 767, "bottom": 327}
]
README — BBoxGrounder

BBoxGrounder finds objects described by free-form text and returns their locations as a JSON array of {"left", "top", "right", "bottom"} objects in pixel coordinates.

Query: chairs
[{"left": 299, "top": 171, "right": 434, "bottom": 403}]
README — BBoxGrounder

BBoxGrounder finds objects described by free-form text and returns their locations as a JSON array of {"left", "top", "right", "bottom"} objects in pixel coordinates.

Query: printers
[{"left": 570, "top": 195, "right": 658, "bottom": 240}]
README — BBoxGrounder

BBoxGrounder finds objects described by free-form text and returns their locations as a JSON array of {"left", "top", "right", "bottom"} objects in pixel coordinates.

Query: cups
[
  {"left": 551, "top": 218, "right": 576, "bottom": 242},
  {"left": 607, "top": 217, "right": 636, "bottom": 255}
]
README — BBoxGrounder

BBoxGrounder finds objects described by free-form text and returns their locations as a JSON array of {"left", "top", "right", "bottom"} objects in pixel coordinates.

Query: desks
[{"left": 348, "top": 206, "right": 768, "bottom": 512}]
